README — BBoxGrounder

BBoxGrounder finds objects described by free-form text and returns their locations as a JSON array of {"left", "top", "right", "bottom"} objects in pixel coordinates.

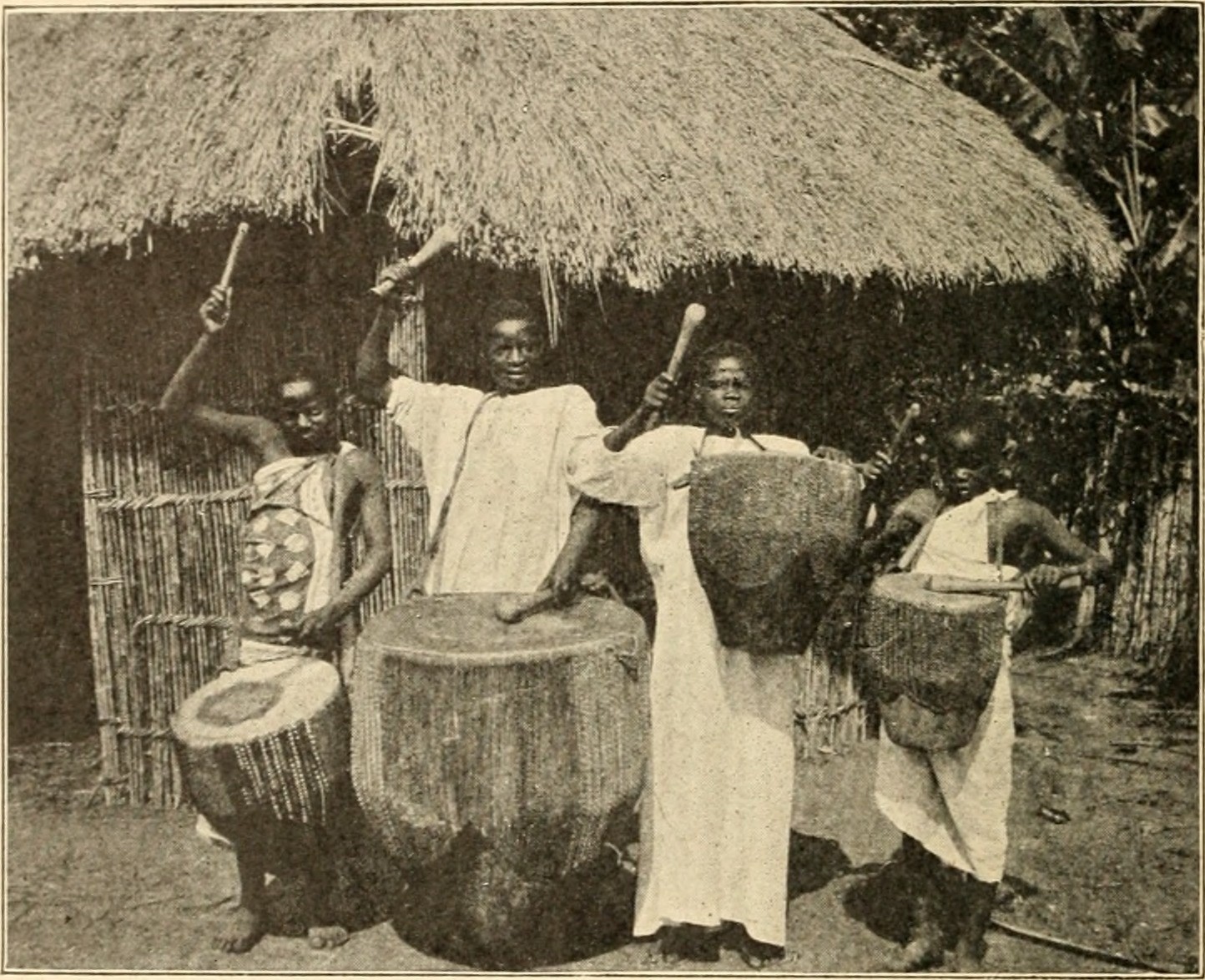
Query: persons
[
  {"left": 857, "top": 400, "right": 1109, "bottom": 972},
  {"left": 356, "top": 257, "right": 608, "bottom": 605},
  {"left": 156, "top": 283, "right": 392, "bottom": 954},
  {"left": 566, "top": 340, "right": 856, "bottom": 968}
]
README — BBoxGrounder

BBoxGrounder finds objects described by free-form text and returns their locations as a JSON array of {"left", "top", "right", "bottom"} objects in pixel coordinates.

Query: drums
[
  {"left": 352, "top": 591, "right": 653, "bottom": 972},
  {"left": 854, "top": 570, "right": 1005, "bottom": 751},
  {"left": 168, "top": 657, "right": 353, "bottom": 868},
  {"left": 688, "top": 454, "right": 862, "bottom": 657}
]
